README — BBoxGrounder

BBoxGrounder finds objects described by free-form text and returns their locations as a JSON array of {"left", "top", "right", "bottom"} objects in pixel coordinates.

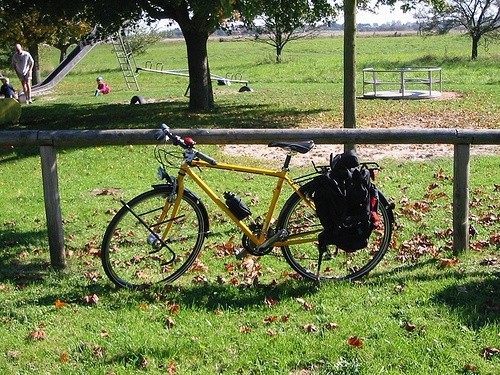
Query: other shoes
[{"left": 25, "top": 100, "right": 32, "bottom": 105}]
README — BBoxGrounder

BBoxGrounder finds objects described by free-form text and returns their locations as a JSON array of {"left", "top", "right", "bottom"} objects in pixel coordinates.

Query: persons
[
  {"left": 93, "top": 76, "right": 110, "bottom": 97},
  {"left": 0, "top": 76, "right": 17, "bottom": 100},
  {"left": 12, "top": 43, "right": 34, "bottom": 104}
]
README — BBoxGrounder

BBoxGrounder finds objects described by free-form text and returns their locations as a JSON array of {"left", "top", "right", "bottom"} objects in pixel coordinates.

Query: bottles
[{"left": 223, "top": 190, "right": 250, "bottom": 220}]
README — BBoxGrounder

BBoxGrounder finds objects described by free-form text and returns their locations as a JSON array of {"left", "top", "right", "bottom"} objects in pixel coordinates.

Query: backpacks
[{"left": 314, "top": 169, "right": 372, "bottom": 251}]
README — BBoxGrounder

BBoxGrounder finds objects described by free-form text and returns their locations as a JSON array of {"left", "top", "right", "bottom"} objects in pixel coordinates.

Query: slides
[{"left": 17, "top": 36, "right": 112, "bottom": 102}]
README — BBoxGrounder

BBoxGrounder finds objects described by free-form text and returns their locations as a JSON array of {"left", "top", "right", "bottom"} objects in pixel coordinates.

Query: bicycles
[{"left": 102, "top": 124, "right": 395, "bottom": 288}]
